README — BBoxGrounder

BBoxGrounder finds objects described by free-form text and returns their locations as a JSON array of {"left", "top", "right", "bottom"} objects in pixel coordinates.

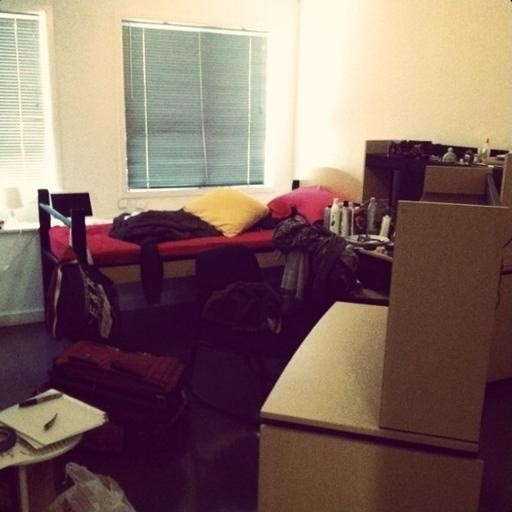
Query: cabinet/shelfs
[{"left": 260, "top": 139, "right": 511, "bottom": 511}]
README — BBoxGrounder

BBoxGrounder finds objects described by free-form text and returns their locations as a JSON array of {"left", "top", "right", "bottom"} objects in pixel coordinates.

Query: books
[{"left": 0, "top": 388, "right": 108, "bottom": 451}]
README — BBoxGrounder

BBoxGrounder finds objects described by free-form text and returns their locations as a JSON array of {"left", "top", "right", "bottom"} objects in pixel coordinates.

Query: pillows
[{"left": 178, "top": 186, "right": 354, "bottom": 239}]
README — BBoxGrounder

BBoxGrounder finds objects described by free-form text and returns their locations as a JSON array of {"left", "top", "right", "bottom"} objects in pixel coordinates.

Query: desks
[{"left": 0, "top": 408, "right": 85, "bottom": 512}]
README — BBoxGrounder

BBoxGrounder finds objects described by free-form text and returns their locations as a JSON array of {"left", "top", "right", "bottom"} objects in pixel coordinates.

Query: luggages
[
  {"left": 30, "top": 338, "right": 189, "bottom": 451},
  {"left": 191, "top": 279, "right": 282, "bottom": 422}
]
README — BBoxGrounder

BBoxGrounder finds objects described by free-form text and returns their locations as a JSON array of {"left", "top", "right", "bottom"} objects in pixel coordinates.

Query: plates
[{"left": 344, "top": 234, "right": 390, "bottom": 246}]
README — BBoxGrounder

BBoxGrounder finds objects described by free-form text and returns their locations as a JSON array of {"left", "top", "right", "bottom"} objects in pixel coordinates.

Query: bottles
[
  {"left": 323, "top": 196, "right": 392, "bottom": 238},
  {"left": 463, "top": 149, "right": 474, "bottom": 167},
  {"left": 477, "top": 138, "right": 492, "bottom": 161},
  {"left": 441, "top": 147, "right": 458, "bottom": 162}
]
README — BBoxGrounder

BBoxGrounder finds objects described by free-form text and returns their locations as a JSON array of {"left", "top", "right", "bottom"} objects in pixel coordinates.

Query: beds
[{"left": 37, "top": 179, "right": 364, "bottom": 339}]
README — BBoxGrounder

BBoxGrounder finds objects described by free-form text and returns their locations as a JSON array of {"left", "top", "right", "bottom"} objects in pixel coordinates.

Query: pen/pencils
[{"left": 19, "top": 393, "right": 62, "bottom": 407}]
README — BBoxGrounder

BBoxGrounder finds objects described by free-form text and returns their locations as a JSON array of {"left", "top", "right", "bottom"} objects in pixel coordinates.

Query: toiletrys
[{"left": 324, "top": 198, "right": 356, "bottom": 238}]
